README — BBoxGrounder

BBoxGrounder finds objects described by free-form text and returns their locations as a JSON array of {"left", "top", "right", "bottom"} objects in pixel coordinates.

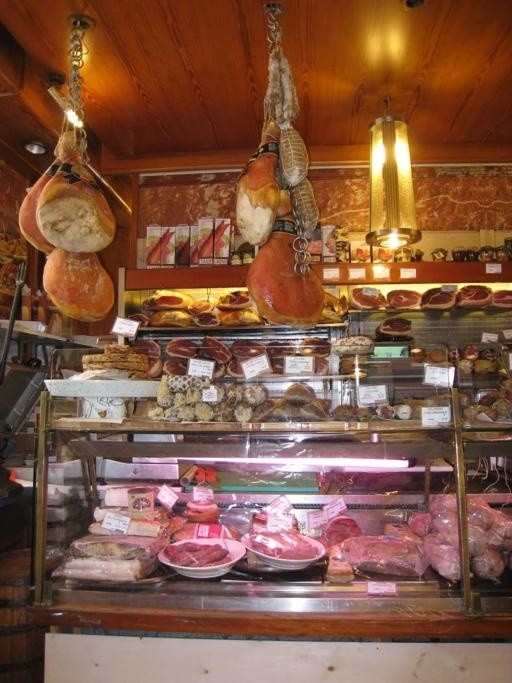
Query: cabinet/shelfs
[{"left": 31, "top": 261, "right": 512, "bottom": 637}]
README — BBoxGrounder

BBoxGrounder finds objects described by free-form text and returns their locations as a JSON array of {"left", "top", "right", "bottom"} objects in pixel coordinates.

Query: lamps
[{"left": 366, "top": 96, "right": 421, "bottom": 248}]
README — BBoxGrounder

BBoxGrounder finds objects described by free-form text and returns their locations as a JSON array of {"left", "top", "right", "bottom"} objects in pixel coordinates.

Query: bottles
[
  {"left": 451, "top": 245, "right": 509, "bottom": 262},
  {"left": 21, "top": 284, "right": 32, "bottom": 321}
]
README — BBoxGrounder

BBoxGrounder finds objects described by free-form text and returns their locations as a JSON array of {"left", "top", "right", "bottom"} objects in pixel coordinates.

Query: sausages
[{"left": 164, "top": 542, "right": 231, "bottom": 567}]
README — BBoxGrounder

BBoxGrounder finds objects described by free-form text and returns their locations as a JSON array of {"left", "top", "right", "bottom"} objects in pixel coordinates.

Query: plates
[
  {"left": 157, "top": 538, "right": 246, "bottom": 578},
  {"left": 241, "top": 532, "right": 326, "bottom": 571}
]
